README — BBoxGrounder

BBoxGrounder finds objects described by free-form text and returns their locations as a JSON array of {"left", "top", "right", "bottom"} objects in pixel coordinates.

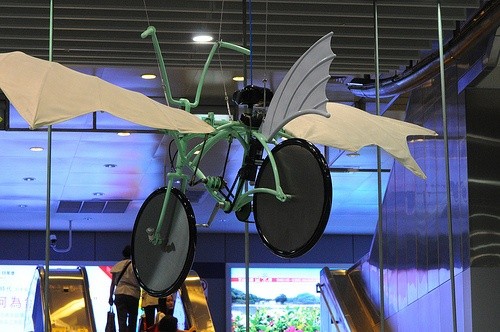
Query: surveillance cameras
[{"left": 50, "top": 234, "right": 57, "bottom": 245}]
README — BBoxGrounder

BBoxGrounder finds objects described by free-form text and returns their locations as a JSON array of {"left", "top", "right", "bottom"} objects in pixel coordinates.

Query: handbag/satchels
[
  {"left": 138, "top": 310, "right": 147, "bottom": 332},
  {"left": 104, "top": 304, "right": 116, "bottom": 332}
]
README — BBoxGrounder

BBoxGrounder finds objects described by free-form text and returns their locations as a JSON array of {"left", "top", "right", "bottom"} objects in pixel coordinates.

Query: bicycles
[{"left": 129, "top": 26, "right": 333, "bottom": 299}]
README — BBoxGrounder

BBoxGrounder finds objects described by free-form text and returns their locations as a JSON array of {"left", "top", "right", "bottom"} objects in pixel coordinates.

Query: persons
[
  {"left": 109, "top": 247, "right": 141, "bottom": 332},
  {"left": 141, "top": 288, "right": 196, "bottom": 332}
]
